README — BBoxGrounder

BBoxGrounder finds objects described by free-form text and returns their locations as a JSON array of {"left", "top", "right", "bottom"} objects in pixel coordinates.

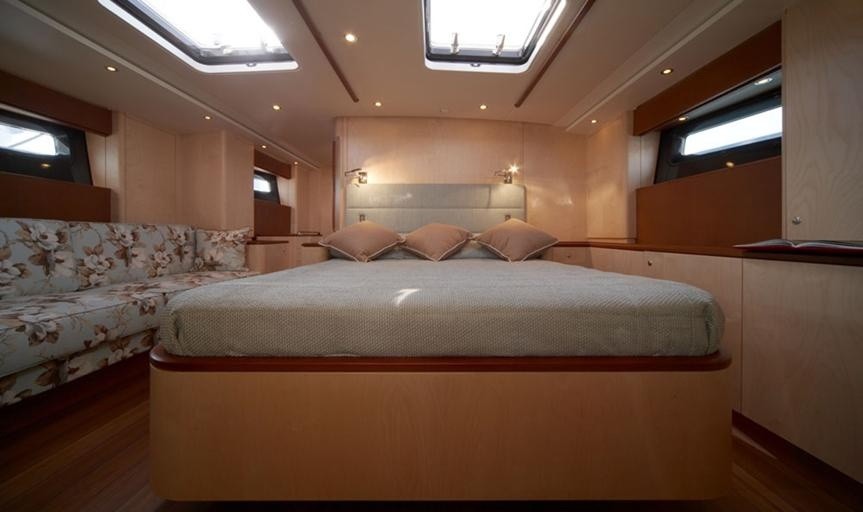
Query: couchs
[{"left": 0, "top": 212, "right": 253, "bottom": 411}]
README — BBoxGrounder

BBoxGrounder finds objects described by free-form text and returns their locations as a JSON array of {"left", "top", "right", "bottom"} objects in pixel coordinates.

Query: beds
[{"left": 147, "top": 181, "right": 734, "bottom": 503}]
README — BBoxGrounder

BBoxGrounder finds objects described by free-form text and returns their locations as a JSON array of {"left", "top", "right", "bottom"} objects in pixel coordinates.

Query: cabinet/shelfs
[{"left": 781, "top": 3, "right": 863, "bottom": 243}]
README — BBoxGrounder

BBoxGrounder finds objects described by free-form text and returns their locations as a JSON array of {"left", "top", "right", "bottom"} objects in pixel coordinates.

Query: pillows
[
  {"left": 317, "top": 216, "right": 561, "bottom": 262},
  {"left": 196, "top": 228, "right": 250, "bottom": 273}
]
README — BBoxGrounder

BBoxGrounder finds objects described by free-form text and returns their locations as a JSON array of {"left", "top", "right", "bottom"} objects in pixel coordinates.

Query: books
[{"left": 731, "top": 238, "right": 863, "bottom": 252}]
